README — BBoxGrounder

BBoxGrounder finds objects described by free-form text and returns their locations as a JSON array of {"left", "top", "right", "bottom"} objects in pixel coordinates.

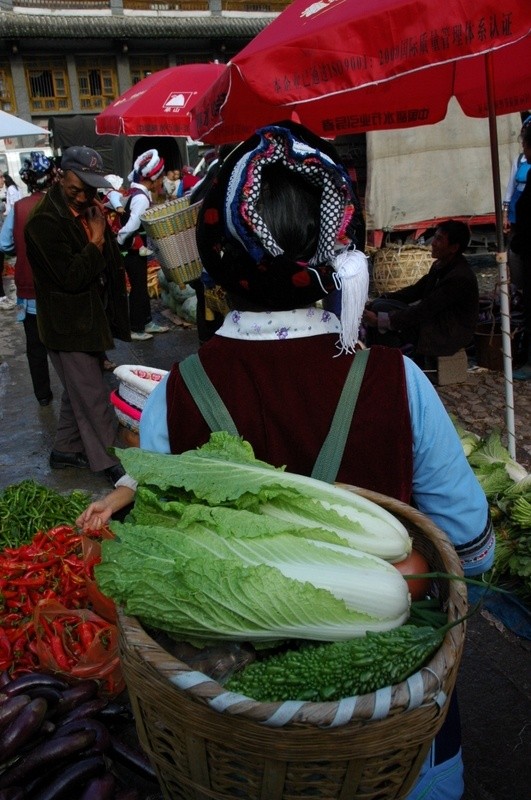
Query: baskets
[
  {"left": 139, "top": 194, "right": 204, "bottom": 285},
  {"left": 113, "top": 482, "right": 466, "bottom": 800}
]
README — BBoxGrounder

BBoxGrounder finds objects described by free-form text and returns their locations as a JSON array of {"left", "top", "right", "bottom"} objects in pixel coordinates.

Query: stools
[{"left": 437, "top": 350, "right": 468, "bottom": 385}]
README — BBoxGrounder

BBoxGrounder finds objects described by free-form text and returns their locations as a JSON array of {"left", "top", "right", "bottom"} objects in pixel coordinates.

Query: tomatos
[{"left": 390, "top": 549, "right": 431, "bottom": 600}]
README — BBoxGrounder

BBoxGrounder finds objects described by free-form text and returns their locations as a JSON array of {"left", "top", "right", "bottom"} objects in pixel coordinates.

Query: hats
[{"left": 61, "top": 146, "right": 115, "bottom": 188}]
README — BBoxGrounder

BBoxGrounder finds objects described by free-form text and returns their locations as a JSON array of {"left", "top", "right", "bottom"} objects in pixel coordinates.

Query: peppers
[{"left": 0, "top": 476, "right": 129, "bottom": 698}]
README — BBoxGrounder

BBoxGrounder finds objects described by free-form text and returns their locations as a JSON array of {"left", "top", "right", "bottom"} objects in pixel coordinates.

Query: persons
[{"left": 0, "top": 114, "right": 531, "bottom": 800}]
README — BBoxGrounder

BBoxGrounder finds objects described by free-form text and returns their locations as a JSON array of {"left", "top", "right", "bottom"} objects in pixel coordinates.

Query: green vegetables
[{"left": 450, "top": 415, "right": 531, "bottom": 596}]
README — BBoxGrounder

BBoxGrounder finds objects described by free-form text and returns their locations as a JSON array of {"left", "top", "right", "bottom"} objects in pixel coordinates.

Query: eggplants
[{"left": 0, "top": 669, "right": 164, "bottom": 800}]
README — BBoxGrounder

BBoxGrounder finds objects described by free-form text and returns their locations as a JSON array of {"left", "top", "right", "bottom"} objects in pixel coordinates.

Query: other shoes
[
  {"left": 131, "top": 330, "right": 153, "bottom": 341},
  {"left": 139, "top": 246, "right": 153, "bottom": 256},
  {"left": 40, "top": 393, "right": 53, "bottom": 406},
  {"left": 104, "top": 359, "right": 117, "bottom": 370},
  {"left": 145, "top": 321, "right": 169, "bottom": 333},
  {"left": 0, "top": 296, "right": 17, "bottom": 309}
]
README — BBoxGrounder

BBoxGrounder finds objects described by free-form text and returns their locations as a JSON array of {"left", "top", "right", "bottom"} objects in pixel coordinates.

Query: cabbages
[{"left": 91, "top": 430, "right": 414, "bottom": 642}]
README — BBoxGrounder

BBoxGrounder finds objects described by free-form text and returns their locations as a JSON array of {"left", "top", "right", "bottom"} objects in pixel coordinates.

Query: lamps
[
  {"left": 12, "top": 41, "right": 18, "bottom": 53},
  {"left": 219, "top": 43, "right": 227, "bottom": 53},
  {"left": 121, "top": 42, "right": 129, "bottom": 52}
]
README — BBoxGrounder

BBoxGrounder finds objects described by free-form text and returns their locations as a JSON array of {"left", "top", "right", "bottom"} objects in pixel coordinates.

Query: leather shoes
[
  {"left": 104, "top": 464, "right": 126, "bottom": 489},
  {"left": 50, "top": 449, "right": 89, "bottom": 469}
]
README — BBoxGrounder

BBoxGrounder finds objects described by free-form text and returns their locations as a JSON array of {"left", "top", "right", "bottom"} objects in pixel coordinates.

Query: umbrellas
[{"left": 0, "top": 0, "right": 531, "bottom": 461}]
